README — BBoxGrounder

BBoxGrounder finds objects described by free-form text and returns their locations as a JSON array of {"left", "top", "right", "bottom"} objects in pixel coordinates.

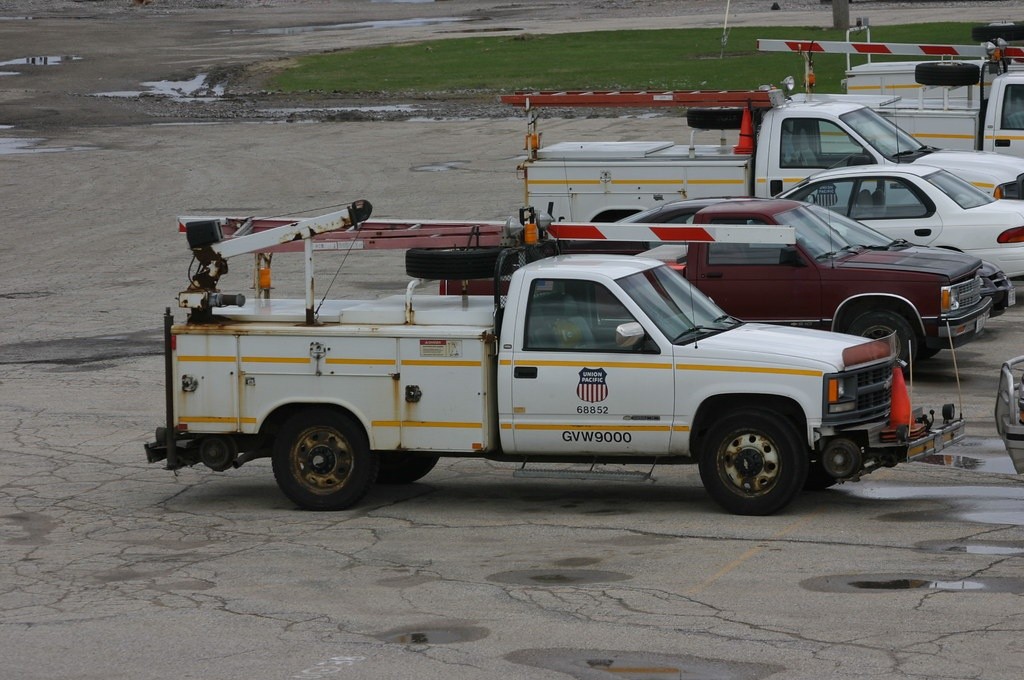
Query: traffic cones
[{"left": 732, "top": 106, "right": 756, "bottom": 155}]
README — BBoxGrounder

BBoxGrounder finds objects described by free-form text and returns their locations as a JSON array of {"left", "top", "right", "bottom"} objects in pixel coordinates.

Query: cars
[
  {"left": 610, "top": 195, "right": 1017, "bottom": 319},
  {"left": 747, "top": 162, "right": 1024, "bottom": 279}
]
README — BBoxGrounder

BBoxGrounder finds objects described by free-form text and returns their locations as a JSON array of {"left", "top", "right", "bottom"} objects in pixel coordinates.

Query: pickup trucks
[{"left": 438, "top": 193, "right": 994, "bottom": 378}]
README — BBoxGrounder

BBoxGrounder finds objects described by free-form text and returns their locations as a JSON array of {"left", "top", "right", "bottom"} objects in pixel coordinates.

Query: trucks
[
  {"left": 515, "top": 88, "right": 1024, "bottom": 223},
  {"left": 755, "top": 37, "right": 1024, "bottom": 160},
  {"left": 143, "top": 192, "right": 968, "bottom": 516}
]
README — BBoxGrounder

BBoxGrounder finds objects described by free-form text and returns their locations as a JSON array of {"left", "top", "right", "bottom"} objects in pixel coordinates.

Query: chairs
[
  {"left": 780, "top": 128, "right": 819, "bottom": 166},
  {"left": 858, "top": 188, "right": 886, "bottom": 217},
  {"left": 528, "top": 295, "right": 596, "bottom": 348}
]
